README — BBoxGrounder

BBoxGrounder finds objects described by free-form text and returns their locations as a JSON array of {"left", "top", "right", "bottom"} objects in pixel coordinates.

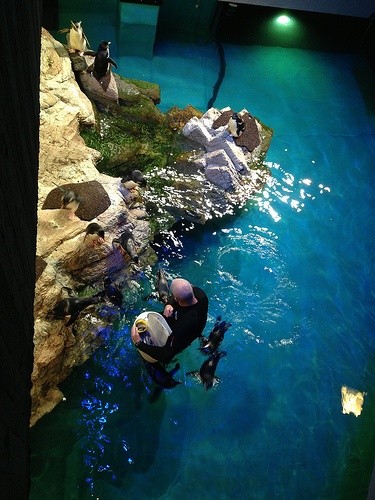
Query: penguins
[
  {"left": 228, "top": 112, "right": 246, "bottom": 138},
  {"left": 56, "top": 20, "right": 92, "bottom": 57},
  {"left": 83, "top": 223, "right": 105, "bottom": 240},
  {"left": 185, "top": 349, "right": 228, "bottom": 394},
  {"left": 51, "top": 180, "right": 87, "bottom": 215},
  {"left": 113, "top": 230, "right": 139, "bottom": 266},
  {"left": 52, "top": 295, "right": 98, "bottom": 327},
  {"left": 83, "top": 40, "right": 118, "bottom": 79},
  {"left": 196, "top": 315, "right": 232, "bottom": 356}
]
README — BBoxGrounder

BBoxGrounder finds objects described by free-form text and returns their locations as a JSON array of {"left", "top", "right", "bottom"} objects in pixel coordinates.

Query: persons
[{"left": 130, "top": 278, "right": 209, "bottom": 366}]
